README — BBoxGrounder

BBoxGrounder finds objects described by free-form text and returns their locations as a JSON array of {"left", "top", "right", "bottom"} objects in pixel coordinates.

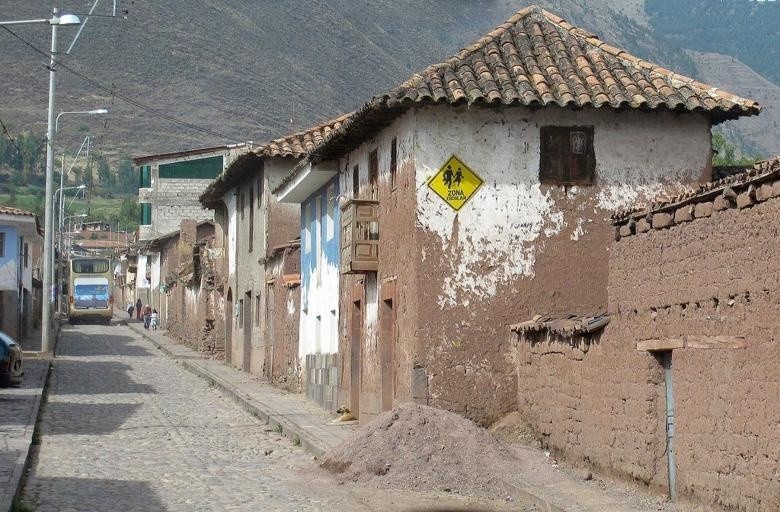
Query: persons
[
  {"left": 136, "top": 298, "right": 142, "bottom": 319},
  {"left": 150, "top": 308, "right": 159, "bottom": 331},
  {"left": 127, "top": 301, "right": 134, "bottom": 320},
  {"left": 143, "top": 304, "right": 151, "bottom": 331}
]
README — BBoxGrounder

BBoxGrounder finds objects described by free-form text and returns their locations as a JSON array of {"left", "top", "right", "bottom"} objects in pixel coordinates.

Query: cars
[{"left": 0, "top": 332, "right": 27, "bottom": 387}]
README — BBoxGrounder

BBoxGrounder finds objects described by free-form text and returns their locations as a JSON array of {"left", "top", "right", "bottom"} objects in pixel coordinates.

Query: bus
[{"left": 66, "top": 257, "right": 115, "bottom": 325}]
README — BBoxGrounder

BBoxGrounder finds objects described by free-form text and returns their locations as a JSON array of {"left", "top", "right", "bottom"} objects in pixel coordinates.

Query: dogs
[{"left": 332, "top": 406, "right": 358, "bottom": 423}]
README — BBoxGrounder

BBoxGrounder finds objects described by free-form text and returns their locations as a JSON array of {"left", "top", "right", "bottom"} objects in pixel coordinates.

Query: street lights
[{"left": 0, "top": 15, "right": 107, "bottom": 353}]
